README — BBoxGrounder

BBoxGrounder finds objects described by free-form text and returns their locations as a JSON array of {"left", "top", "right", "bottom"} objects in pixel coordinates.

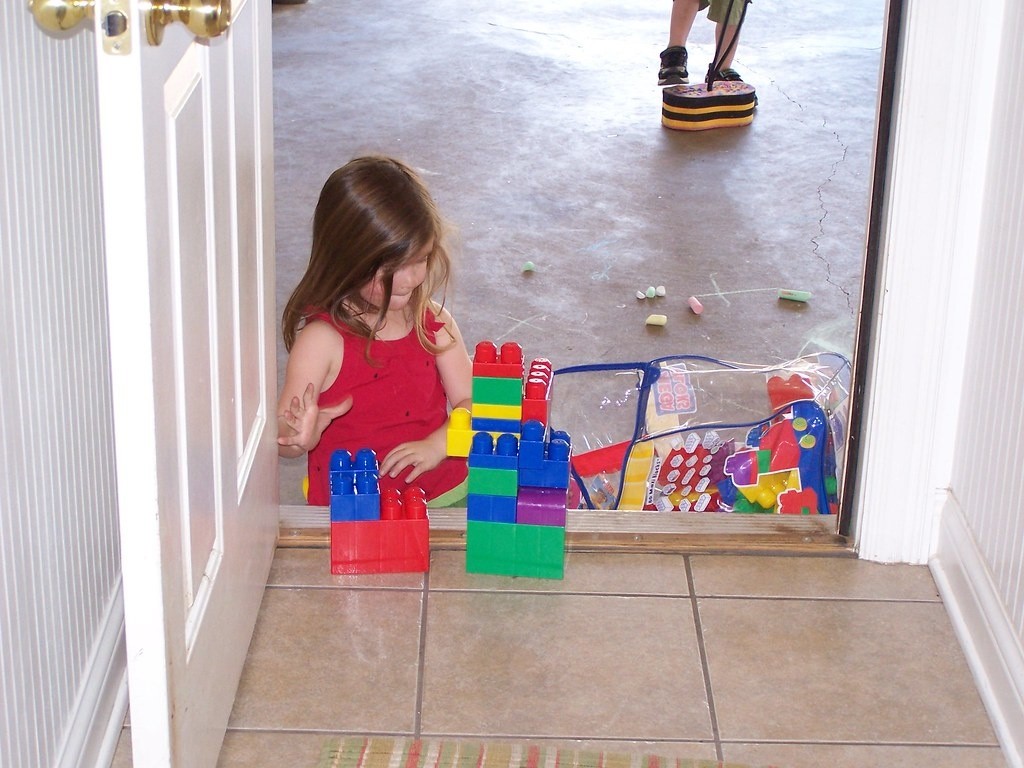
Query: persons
[
  {"left": 276, "top": 155, "right": 474, "bottom": 507},
  {"left": 658, "top": 0, "right": 759, "bottom": 107}
]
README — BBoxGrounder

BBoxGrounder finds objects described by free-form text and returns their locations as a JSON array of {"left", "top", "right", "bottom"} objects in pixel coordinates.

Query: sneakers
[
  {"left": 705, "top": 62, "right": 759, "bottom": 106},
  {"left": 658, "top": 46, "right": 688, "bottom": 85}
]
